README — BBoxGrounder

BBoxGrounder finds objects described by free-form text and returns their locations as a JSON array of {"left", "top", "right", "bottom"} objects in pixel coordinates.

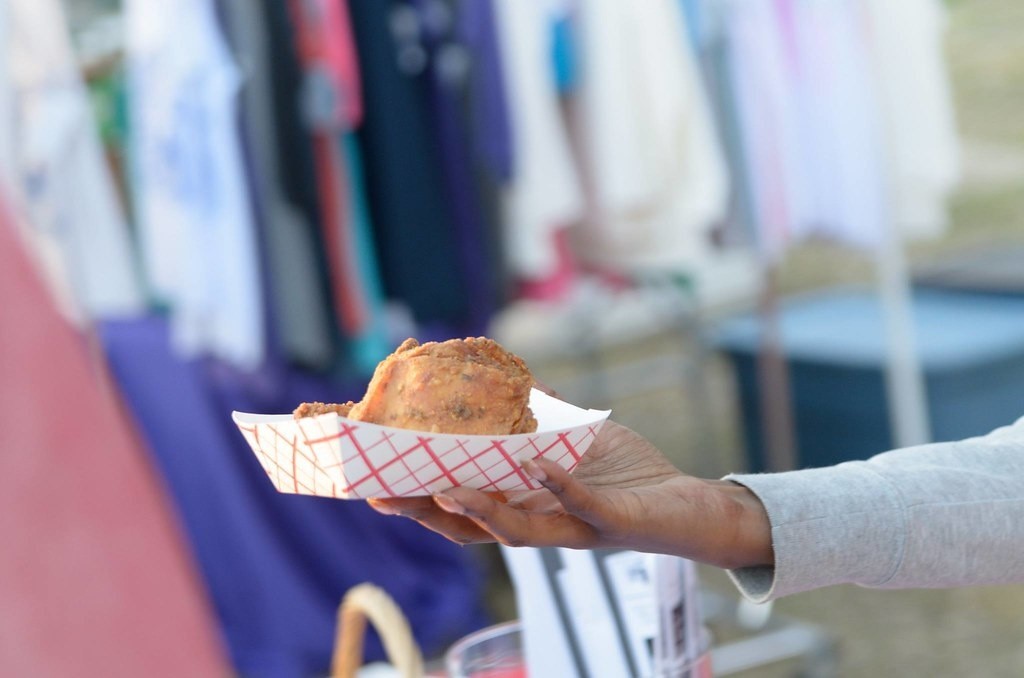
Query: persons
[{"left": 365, "top": 372, "right": 1023, "bottom": 604}]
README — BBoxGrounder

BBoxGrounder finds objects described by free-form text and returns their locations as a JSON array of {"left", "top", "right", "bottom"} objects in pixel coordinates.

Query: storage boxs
[
  {"left": 720, "top": 282, "right": 1024, "bottom": 471},
  {"left": 231, "top": 388, "right": 613, "bottom": 501}
]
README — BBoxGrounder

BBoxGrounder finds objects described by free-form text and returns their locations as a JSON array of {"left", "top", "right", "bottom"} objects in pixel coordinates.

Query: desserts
[{"left": 293, "top": 334, "right": 539, "bottom": 436}]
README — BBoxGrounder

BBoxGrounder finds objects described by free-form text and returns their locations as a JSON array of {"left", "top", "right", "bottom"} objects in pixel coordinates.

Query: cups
[{"left": 447, "top": 618, "right": 716, "bottom": 678}]
